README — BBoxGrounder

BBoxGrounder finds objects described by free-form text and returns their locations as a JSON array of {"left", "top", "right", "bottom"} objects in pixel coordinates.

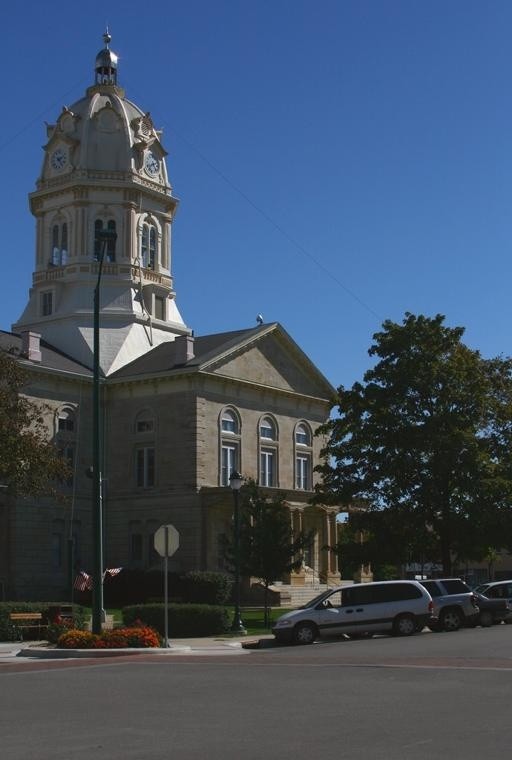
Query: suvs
[{"left": 419, "top": 576, "right": 479, "bottom": 633}]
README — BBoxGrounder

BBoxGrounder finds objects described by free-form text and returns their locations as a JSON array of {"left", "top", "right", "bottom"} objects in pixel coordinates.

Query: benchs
[{"left": 8, "top": 611, "right": 51, "bottom": 642}]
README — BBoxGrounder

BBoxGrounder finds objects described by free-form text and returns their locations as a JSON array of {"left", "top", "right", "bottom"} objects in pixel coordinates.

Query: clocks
[
  {"left": 144, "top": 152, "right": 161, "bottom": 178},
  {"left": 49, "top": 148, "right": 68, "bottom": 172}
]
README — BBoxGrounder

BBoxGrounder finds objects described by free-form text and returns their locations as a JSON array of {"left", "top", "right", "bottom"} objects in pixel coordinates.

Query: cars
[{"left": 474, "top": 590, "right": 510, "bottom": 628}]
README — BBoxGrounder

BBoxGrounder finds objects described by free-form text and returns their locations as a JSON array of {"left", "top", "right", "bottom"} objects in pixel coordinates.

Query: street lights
[
  {"left": 90, "top": 228, "right": 115, "bottom": 636},
  {"left": 228, "top": 470, "right": 249, "bottom": 636}
]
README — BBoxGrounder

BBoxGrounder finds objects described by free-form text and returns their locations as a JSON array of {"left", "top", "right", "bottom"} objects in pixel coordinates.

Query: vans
[
  {"left": 272, "top": 578, "right": 434, "bottom": 644},
  {"left": 475, "top": 579, "right": 512, "bottom": 623}
]
